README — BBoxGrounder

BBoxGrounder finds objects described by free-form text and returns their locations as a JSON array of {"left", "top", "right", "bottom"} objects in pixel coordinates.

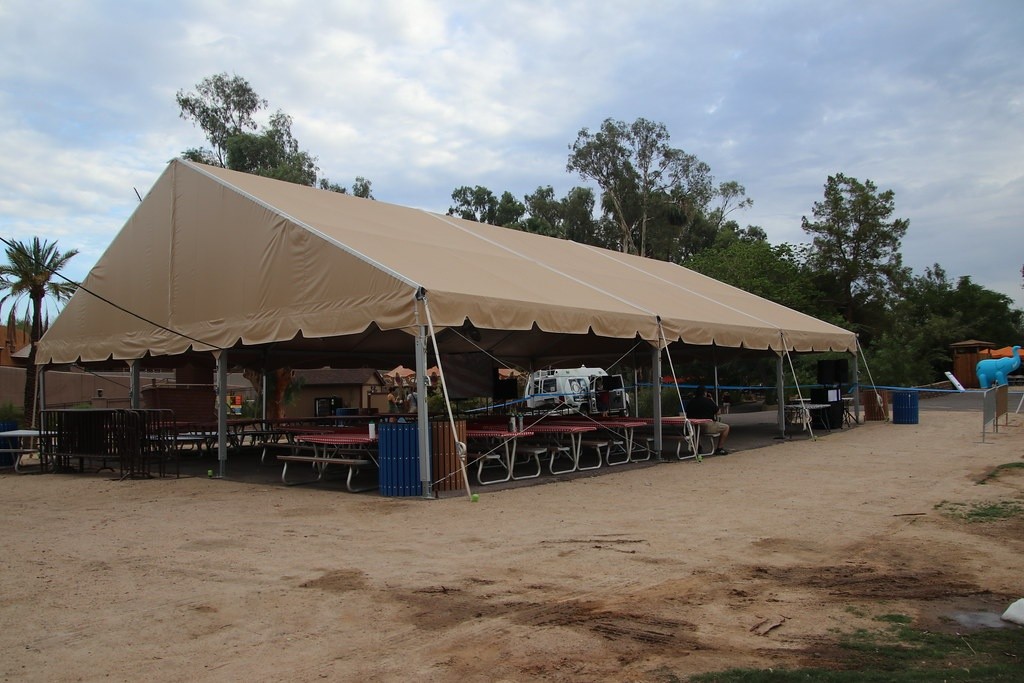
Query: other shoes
[
  {"left": 698, "top": 445, "right": 702, "bottom": 452},
  {"left": 715, "top": 448, "right": 728, "bottom": 455}
]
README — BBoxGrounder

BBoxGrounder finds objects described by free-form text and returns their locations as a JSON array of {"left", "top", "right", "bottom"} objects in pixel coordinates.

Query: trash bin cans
[
  {"left": 892, "top": 390, "right": 918, "bottom": 425},
  {"left": 377, "top": 413, "right": 423, "bottom": 496},
  {"left": 864, "top": 389, "right": 889, "bottom": 421},
  {"left": 430, "top": 412, "right": 468, "bottom": 490}
]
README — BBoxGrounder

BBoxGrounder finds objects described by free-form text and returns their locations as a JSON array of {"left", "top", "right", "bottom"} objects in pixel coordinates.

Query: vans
[{"left": 523, "top": 362, "right": 630, "bottom": 417}]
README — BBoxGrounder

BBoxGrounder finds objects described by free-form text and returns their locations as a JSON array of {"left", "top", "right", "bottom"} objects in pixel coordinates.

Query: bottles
[
  {"left": 369, "top": 421, "right": 375, "bottom": 438},
  {"left": 508, "top": 413, "right": 516, "bottom": 432},
  {"left": 518, "top": 414, "right": 523, "bottom": 431}
]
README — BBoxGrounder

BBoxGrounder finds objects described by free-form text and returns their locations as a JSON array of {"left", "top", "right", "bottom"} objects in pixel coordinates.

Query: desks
[
  {"left": 785, "top": 404, "right": 831, "bottom": 441},
  {"left": 0, "top": 416, "right": 713, "bottom": 492},
  {"left": 795, "top": 397, "right": 853, "bottom": 423}
]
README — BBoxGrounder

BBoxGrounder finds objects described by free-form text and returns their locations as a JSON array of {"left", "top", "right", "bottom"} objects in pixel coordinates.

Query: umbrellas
[
  {"left": 979, "top": 346, "right": 1024, "bottom": 358},
  {"left": 382, "top": 365, "right": 520, "bottom": 382}
]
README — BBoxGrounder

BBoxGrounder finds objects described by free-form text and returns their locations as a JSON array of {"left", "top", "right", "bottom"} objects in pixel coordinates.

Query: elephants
[{"left": 976, "top": 346, "right": 1021, "bottom": 389}]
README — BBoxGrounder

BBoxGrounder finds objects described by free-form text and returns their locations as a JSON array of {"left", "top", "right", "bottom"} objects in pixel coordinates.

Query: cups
[
  {"left": 208, "top": 468, "right": 213, "bottom": 477},
  {"left": 471, "top": 491, "right": 479, "bottom": 501},
  {"left": 679, "top": 412, "right": 687, "bottom": 417}
]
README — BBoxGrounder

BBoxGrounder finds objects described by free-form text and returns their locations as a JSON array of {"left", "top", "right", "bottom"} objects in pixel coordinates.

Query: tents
[{"left": 11, "top": 159, "right": 859, "bottom": 502}]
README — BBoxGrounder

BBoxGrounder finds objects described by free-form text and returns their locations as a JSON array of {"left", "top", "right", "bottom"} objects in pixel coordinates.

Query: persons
[
  {"left": 387, "top": 387, "right": 399, "bottom": 423},
  {"left": 407, "top": 388, "right": 419, "bottom": 416},
  {"left": 685, "top": 386, "right": 729, "bottom": 455},
  {"left": 601, "top": 387, "right": 609, "bottom": 417}
]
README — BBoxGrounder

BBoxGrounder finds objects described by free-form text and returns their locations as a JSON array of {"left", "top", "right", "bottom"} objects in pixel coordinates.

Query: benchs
[{"left": 0, "top": 430, "right": 724, "bottom": 492}]
